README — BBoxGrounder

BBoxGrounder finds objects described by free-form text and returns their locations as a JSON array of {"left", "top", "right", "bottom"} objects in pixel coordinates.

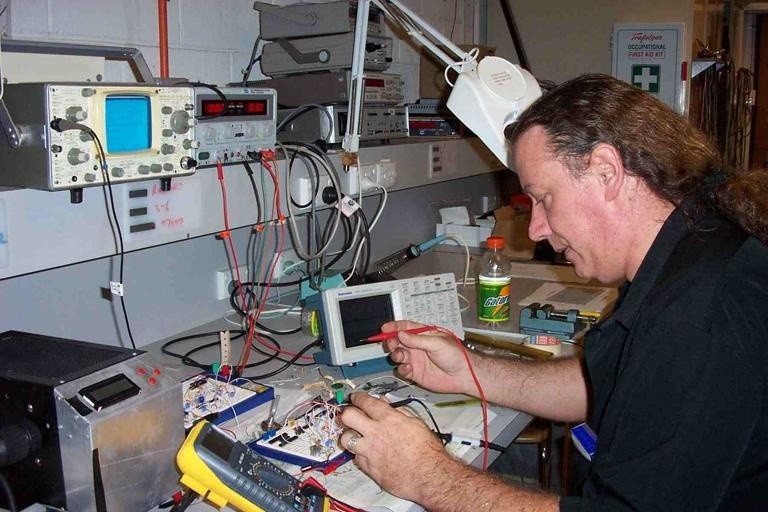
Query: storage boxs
[{"left": 420, "top": 44, "right": 497, "bottom": 99}]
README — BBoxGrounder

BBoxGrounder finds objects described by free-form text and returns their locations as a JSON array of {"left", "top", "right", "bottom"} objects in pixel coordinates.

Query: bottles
[{"left": 478, "top": 235, "right": 513, "bottom": 329}]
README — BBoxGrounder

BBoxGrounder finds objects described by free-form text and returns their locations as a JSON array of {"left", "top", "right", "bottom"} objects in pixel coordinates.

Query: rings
[{"left": 348, "top": 430, "right": 361, "bottom": 454}]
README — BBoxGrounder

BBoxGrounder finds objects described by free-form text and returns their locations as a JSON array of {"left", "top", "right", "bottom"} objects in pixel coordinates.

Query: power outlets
[
  {"left": 375, "top": 162, "right": 396, "bottom": 190},
  {"left": 217, "top": 266, "right": 249, "bottom": 300},
  {"left": 272, "top": 248, "right": 302, "bottom": 280},
  {"left": 297, "top": 175, "right": 333, "bottom": 210},
  {"left": 350, "top": 164, "right": 378, "bottom": 196}
]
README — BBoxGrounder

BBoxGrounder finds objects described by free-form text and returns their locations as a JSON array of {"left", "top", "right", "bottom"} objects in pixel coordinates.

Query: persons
[{"left": 339, "top": 74, "right": 767, "bottom": 510}]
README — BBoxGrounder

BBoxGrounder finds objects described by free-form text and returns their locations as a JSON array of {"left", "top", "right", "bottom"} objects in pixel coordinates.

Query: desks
[{"left": 151, "top": 239, "right": 627, "bottom": 512}]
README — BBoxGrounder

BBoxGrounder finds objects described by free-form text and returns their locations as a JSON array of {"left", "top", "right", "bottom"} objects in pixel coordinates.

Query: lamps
[{"left": 342, "top": 0, "right": 543, "bottom": 173}]
